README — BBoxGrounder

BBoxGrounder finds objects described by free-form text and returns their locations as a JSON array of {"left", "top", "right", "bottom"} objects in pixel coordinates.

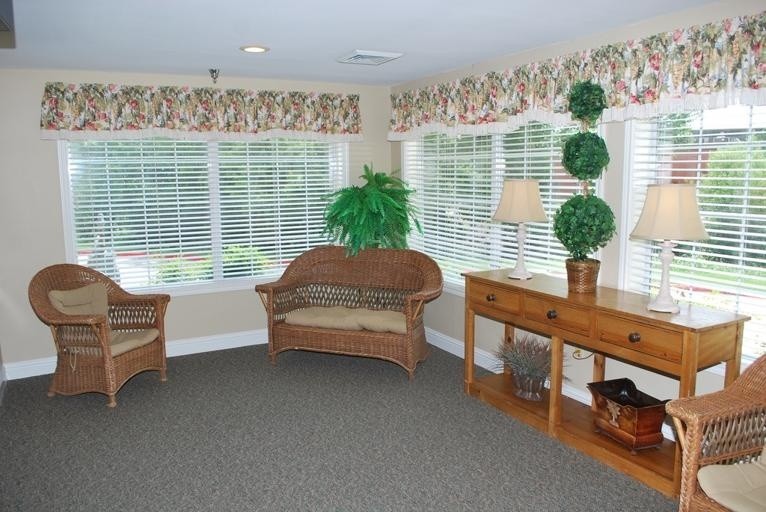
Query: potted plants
[
  {"left": 493, "top": 334, "right": 552, "bottom": 402},
  {"left": 553, "top": 79, "right": 616, "bottom": 294}
]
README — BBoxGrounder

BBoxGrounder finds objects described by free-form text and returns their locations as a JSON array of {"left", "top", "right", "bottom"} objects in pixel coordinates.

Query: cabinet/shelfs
[{"left": 460, "top": 266, "right": 751, "bottom": 500}]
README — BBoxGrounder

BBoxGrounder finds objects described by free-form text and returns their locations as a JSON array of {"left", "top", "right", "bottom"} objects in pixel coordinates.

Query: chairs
[
  {"left": 28, "top": 263, "right": 170, "bottom": 407},
  {"left": 664, "top": 354, "right": 765, "bottom": 511}
]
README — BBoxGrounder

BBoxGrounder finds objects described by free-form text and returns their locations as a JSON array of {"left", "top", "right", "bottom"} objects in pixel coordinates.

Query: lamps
[
  {"left": 492, "top": 179, "right": 549, "bottom": 279},
  {"left": 628, "top": 184, "right": 710, "bottom": 313}
]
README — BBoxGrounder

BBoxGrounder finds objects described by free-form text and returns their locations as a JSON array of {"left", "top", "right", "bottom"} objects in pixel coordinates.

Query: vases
[{"left": 586, "top": 377, "right": 671, "bottom": 456}]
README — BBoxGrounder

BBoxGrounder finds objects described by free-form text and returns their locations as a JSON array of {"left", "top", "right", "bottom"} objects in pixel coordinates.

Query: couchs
[{"left": 255, "top": 246, "right": 443, "bottom": 381}]
